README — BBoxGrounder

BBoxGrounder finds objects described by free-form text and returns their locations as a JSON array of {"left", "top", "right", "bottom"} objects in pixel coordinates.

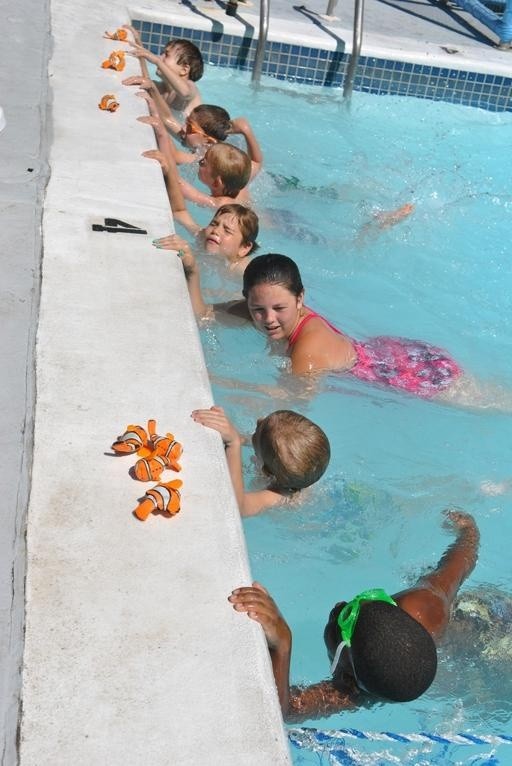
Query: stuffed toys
[
  {"left": 104, "top": 29, "right": 130, "bottom": 43},
  {"left": 101, "top": 49, "right": 127, "bottom": 72},
  {"left": 111, "top": 419, "right": 183, "bottom": 521},
  {"left": 99, "top": 94, "right": 122, "bottom": 112}
]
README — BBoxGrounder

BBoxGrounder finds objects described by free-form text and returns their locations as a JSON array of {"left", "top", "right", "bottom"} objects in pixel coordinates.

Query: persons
[
  {"left": 227, "top": 506, "right": 512, "bottom": 728},
  {"left": 124, "top": 74, "right": 234, "bottom": 165},
  {"left": 124, "top": 22, "right": 208, "bottom": 116},
  {"left": 188, "top": 405, "right": 511, "bottom": 519},
  {"left": 134, "top": 115, "right": 414, "bottom": 255},
  {"left": 150, "top": 232, "right": 511, "bottom": 417},
  {"left": 141, "top": 148, "right": 264, "bottom": 302}
]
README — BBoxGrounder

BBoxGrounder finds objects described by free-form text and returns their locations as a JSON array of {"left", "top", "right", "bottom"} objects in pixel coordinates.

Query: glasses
[
  {"left": 328, "top": 588, "right": 398, "bottom": 647},
  {"left": 186, "top": 116, "right": 209, "bottom": 138}
]
